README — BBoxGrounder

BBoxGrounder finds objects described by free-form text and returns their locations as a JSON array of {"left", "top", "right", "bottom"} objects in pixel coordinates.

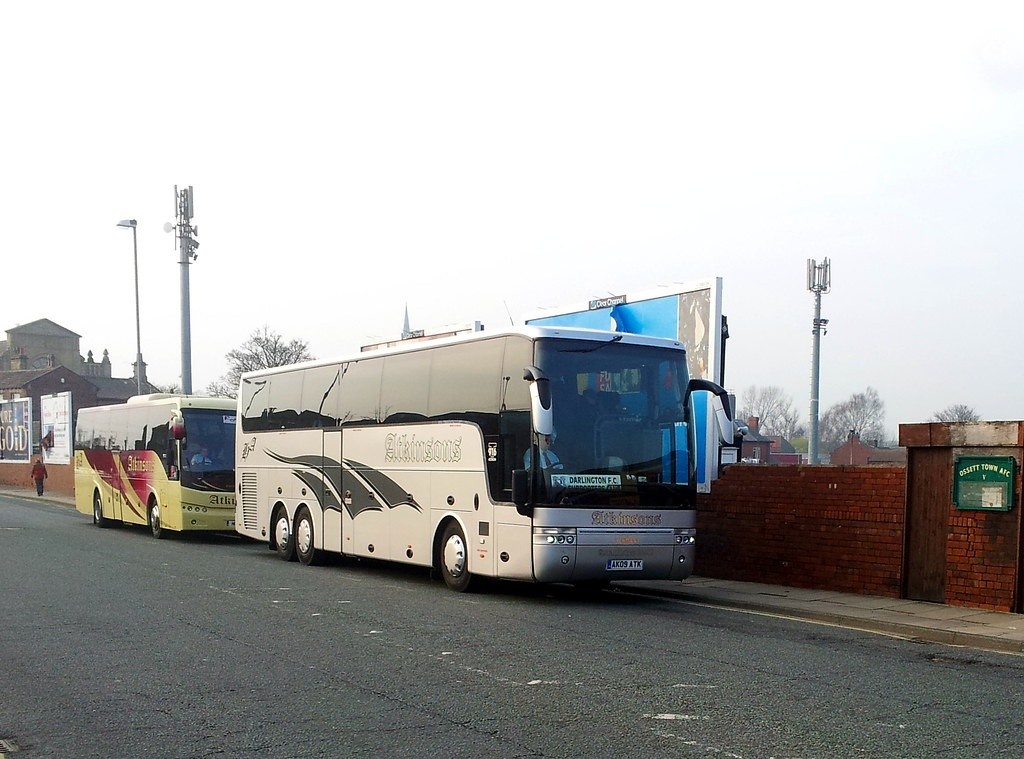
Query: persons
[
  {"left": 191, "top": 448, "right": 211, "bottom": 465},
  {"left": 31, "top": 458, "right": 48, "bottom": 496},
  {"left": 523, "top": 434, "right": 563, "bottom": 471}
]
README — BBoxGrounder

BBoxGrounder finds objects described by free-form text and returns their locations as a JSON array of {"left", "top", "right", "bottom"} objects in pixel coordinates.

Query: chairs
[{"left": 515, "top": 382, "right": 628, "bottom": 471}]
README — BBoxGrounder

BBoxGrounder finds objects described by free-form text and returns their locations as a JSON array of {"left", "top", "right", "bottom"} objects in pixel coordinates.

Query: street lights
[{"left": 116, "top": 219, "right": 141, "bottom": 396}]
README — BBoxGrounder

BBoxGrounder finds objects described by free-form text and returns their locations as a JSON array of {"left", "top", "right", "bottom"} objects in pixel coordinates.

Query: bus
[
  {"left": 73, "top": 393, "right": 237, "bottom": 540},
  {"left": 234, "top": 325, "right": 734, "bottom": 593}
]
